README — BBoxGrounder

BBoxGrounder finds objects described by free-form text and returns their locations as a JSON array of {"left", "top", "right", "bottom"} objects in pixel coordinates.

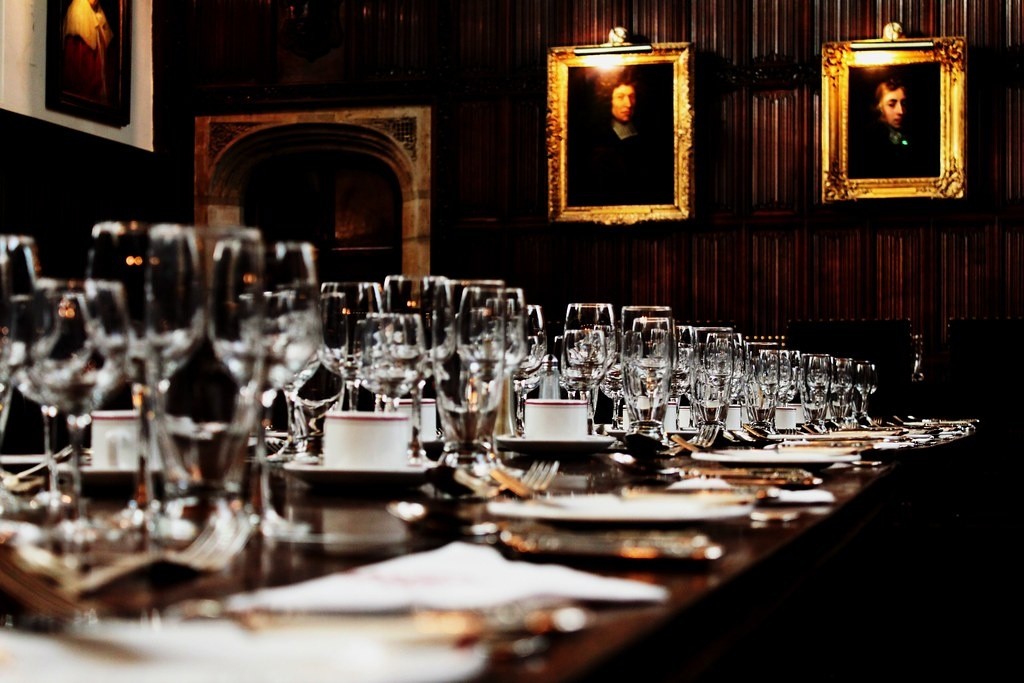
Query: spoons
[
  {"left": 732, "top": 430, "right": 756, "bottom": 442},
  {"left": 743, "top": 424, "right": 765, "bottom": 439}
]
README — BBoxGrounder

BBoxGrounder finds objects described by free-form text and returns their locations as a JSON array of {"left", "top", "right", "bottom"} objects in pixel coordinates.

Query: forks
[
  {"left": 457, "top": 461, "right": 560, "bottom": 517},
  {"left": 658, "top": 424, "right": 720, "bottom": 456},
  {"left": 780, "top": 429, "right": 797, "bottom": 442},
  {"left": 64, "top": 513, "right": 255, "bottom": 597}
]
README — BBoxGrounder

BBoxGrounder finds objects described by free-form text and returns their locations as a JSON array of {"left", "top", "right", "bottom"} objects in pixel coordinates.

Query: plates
[
  {"left": 693, "top": 448, "right": 861, "bottom": 463},
  {"left": 486, "top": 495, "right": 753, "bottom": 524},
  {"left": 903, "top": 421, "right": 967, "bottom": 426},
  {"left": 282, "top": 460, "right": 432, "bottom": 493},
  {"left": 604, "top": 428, "right": 627, "bottom": 435},
  {"left": 76, "top": 467, "right": 164, "bottom": 499},
  {"left": 667, "top": 430, "right": 698, "bottom": 435},
  {"left": 766, "top": 433, "right": 880, "bottom": 439},
  {"left": 495, "top": 433, "right": 617, "bottom": 453}
]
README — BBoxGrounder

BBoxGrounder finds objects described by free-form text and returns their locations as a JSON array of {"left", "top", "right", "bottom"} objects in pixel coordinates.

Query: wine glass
[{"left": 0, "top": 220, "right": 877, "bottom": 549}]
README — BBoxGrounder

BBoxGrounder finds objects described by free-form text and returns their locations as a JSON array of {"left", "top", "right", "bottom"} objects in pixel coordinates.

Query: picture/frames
[
  {"left": 821, "top": 37, "right": 966, "bottom": 200},
  {"left": 547, "top": 40, "right": 693, "bottom": 223}
]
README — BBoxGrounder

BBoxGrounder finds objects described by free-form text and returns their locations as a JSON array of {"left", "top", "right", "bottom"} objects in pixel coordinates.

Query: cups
[
  {"left": 322, "top": 412, "right": 411, "bottom": 468},
  {"left": 787, "top": 403, "right": 804, "bottom": 423},
  {"left": 725, "top": 405, "right": 741, "bottom": 430},
  {"left": 88, "top": 410, "right": 141, "bottom": 469},
  {"left": 663, "top": 403, "right": 678, "bottom": 431},
  {"left": 623, "top": 405, "right": 630, "bottom": 430},
  {"left": 524, "top": 399, "right": 589, "bottom": 440},
  {"left": 775, "top": 406, "right": 796, "bottom": 429},
  {"left": 396, "top": 398, "right": 437, "bottom": 442},
  {"left": 677, "top": 406, "right": 692, "bottom": 428}
]
